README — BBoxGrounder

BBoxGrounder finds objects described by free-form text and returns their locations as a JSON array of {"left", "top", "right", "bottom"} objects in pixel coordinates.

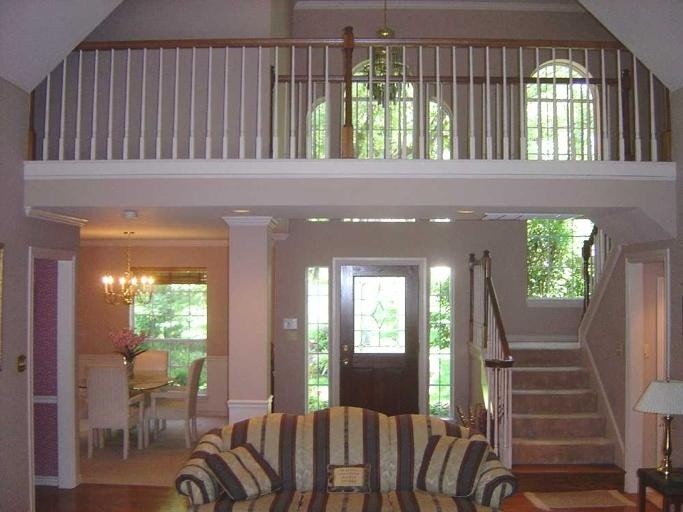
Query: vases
[{"left": 125, "top": 354, "right": 133, "bottom": 379}]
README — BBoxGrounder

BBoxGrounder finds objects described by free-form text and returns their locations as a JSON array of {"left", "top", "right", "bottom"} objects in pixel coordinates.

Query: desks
[
  {"left": 636, "top": 467, "right": 682, "bottom": 512},
  {"left": 76, "top": 374, "right": 174, "bottom": 442}
]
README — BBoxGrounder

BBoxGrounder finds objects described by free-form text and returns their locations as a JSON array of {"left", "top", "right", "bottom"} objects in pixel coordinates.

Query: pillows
[
  {"left": 204, "top": 441, "right": 282, "bottom": 502},
  {"left": 415, "top": 434, "right": 489, "bottom": 500},
  {"left": 325, "top": 463, "right": 371, "bottom": 495}
]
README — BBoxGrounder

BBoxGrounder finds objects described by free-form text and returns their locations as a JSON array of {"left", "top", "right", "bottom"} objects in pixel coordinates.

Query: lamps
[
  {"left": 102, "top": 232, "right": 153, "bottom": 304},
  {"left": 631, "top": 379, "right": 682, "bottom": 475},
  {"left": 363, "top": 0, "right": 409, "bottom": 108}
]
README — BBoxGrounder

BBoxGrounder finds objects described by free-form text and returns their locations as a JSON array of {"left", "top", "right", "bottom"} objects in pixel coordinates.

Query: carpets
[{"left": 522, "top": 488, "right": 637, "bottom": 511}]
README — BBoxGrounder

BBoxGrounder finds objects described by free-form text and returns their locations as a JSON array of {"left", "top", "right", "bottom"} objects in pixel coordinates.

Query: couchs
[{"left": 173, "top": 405, "right": 517, "bottom": 512}]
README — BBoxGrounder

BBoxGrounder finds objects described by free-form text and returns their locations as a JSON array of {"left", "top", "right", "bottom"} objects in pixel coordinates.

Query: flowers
[{"left": 108, "top": 328, "right": 149, "bottom": 362}]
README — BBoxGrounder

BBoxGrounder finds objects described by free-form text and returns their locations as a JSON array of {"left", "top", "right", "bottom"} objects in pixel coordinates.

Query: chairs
[
  {"left": 84, "top": 364, "right": 144, "bottom": 459},
  {"left": 133, "top": 351, "right": 168, "bottom": 433},
  {"left": 144, "top": 358, "right": 204, "bottom": 450}
]
